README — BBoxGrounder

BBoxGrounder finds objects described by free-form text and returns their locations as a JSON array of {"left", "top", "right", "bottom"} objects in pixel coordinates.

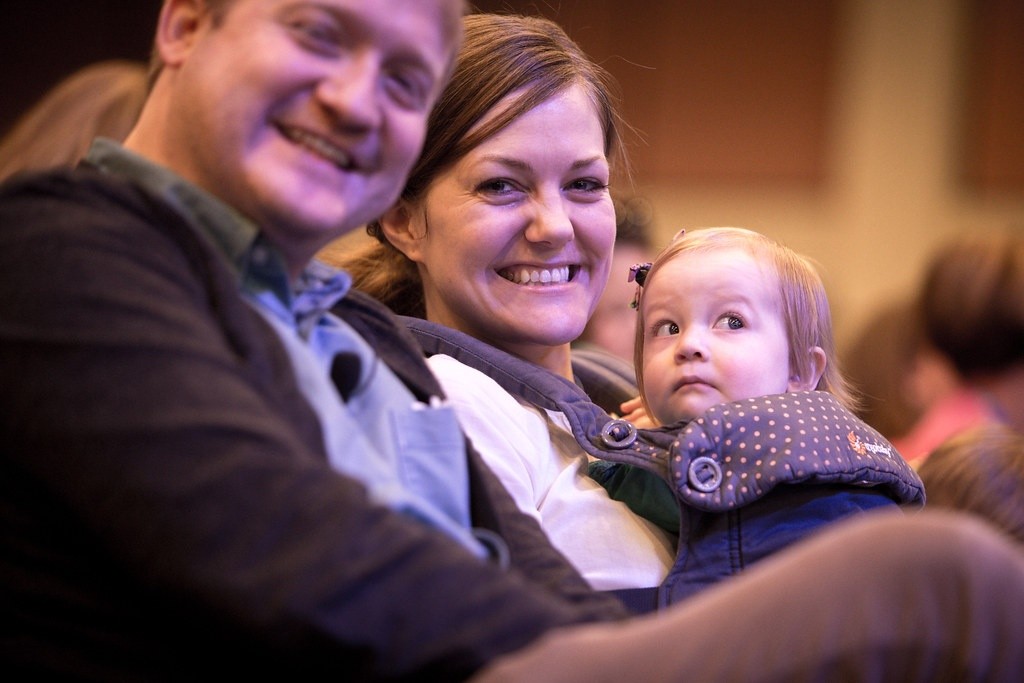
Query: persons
[
  {"left": 840, "top": 219, "right": 1024, "bottom": 557},
  {"left": 0, "top": 56, "right": 149, "bottom": 185},
  {"left": 613, "top": 226, "right": 927, "bottom": 617},
  {"left": 0, "top": 0, "right": 1024, "bottom": 683},
  {"left": 327, "top": 12, "right": 681, "bottom": 618}
]
